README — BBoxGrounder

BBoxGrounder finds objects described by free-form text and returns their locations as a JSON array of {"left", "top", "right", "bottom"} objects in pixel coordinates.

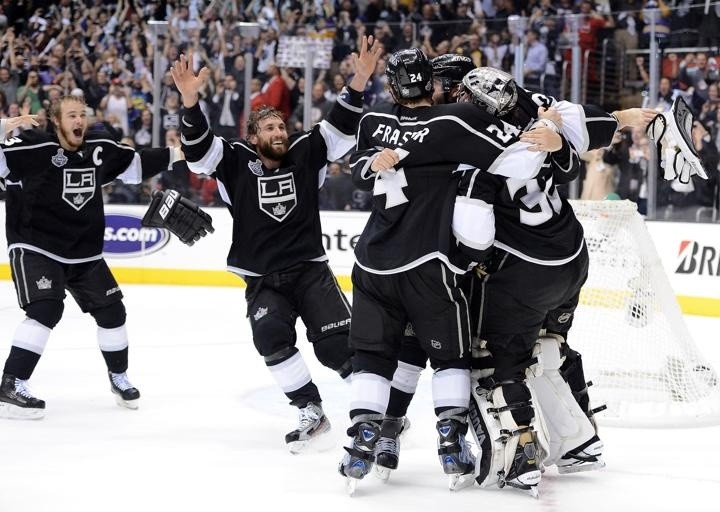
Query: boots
[
  {"left": 554, "top": 433, "right": 605, "bottom": 467},
  {"left": 436, "top": 417, "right": 478, "bottom": 475},
  {"left": 495, "top": 441, "right": 541, "bottom": 489},
  {"left": 0, "top": 373, "right": 45, "bottom": 408},
  {"left": 108, "top": 370, "right": 140, "bottom": 401},
  {"left": 374, "top": 414, "right": 409, "bottom": 469},
  {"left": 284, "top": 392, "right": 329, "bottom": 445},
  {"left": 342, "top": 420, "right": 381, "bottom": 479}
]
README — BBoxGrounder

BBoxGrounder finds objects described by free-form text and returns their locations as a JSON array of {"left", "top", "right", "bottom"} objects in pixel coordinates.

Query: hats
[
  {"left": 524, "top": 26, "right": 542, "bottom": 37},
  {"left": 111, "top": 78, "right": 123, "bottom": 86}
]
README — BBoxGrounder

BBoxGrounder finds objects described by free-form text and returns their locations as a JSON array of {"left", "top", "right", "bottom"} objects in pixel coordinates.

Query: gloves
[
  {"left": 139, "top": 187, "right": 216, "bottom": 248},
  {"left": 644, "top": 95, "right": 710, "bottom": 184}
]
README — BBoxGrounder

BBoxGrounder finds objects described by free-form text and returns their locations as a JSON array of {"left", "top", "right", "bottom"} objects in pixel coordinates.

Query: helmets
[
  {"left": 383, "top": 48, "right": 433, "bottom": 101},
  {"left": 429, "top": 53, "right": 476, "bottom": 86},
  {"left": 456, "top": 66, "right": 519, "bottom": 117}
]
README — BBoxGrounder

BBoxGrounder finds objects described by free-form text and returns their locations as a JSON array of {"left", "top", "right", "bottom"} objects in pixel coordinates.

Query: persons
[
  {"left": 0, "top": 0, "right": 720, "bottom": 223},
  {"left": 337, "top": 46, "right": 659, "bottom": 487},
  {"left": 0, "top": 93, "right": 187, "bottom": 410},
  {"left": 169, "top": 33, "right": 383, "bottom": 444}
]
